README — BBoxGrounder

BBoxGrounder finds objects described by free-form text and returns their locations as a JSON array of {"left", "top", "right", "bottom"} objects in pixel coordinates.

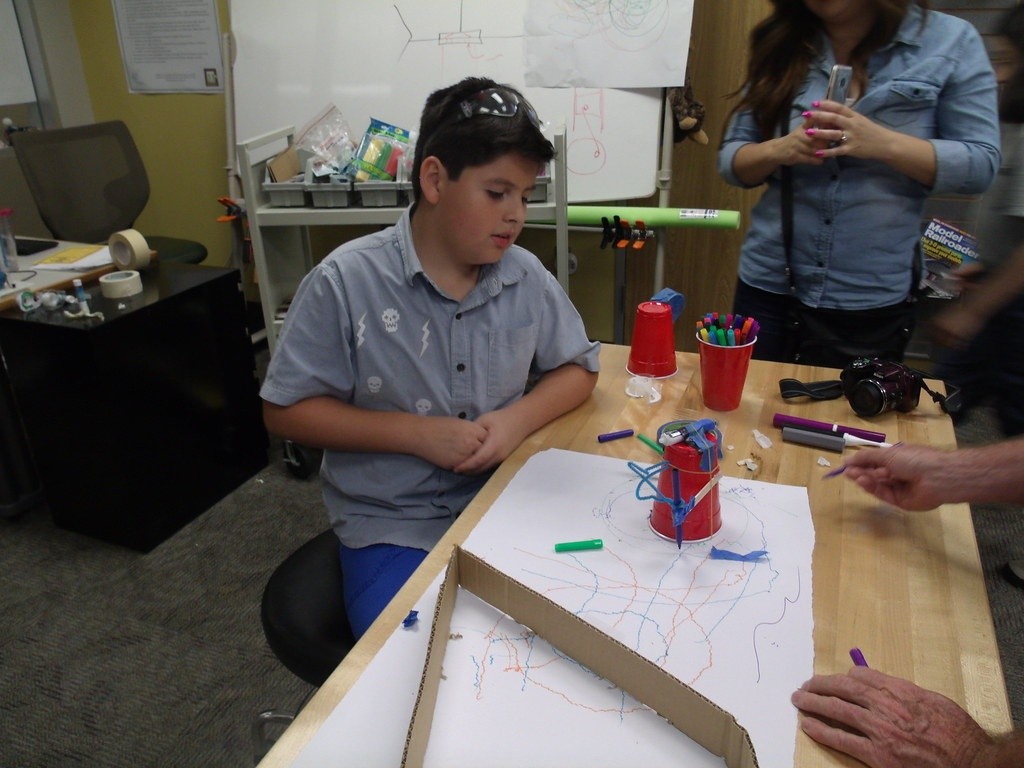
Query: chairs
[{"left": 8, "top": 117, "right": 207, "bottom": 264}]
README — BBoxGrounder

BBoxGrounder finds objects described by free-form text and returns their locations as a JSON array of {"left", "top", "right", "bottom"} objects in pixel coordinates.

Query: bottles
[{"left": 0, "top": 208, "right": 18, "bottom": 289}]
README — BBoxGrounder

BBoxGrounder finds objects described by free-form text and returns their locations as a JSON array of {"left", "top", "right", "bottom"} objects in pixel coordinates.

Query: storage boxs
[{"left": 260, "top": 172, "right": 552, "bottom": 209}]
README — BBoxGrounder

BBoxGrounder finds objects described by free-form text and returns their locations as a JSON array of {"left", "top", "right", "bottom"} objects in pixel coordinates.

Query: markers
[
  {"left": 696, "top": 312, "right": 760, "bottom": 346},
  {"left": 773, "top": 414, "right": 886, "bottom": 443}
]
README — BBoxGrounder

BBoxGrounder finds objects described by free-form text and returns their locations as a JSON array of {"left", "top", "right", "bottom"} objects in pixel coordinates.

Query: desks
[
  {"left": 251, "top": 343, "right": 1013, "bottom": 768},
  {"left": 0, "top": 256, "right": 271, "bottom": 556}
]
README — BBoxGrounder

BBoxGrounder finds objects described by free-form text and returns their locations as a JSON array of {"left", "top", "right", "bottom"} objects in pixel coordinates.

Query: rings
[{"left": 839, "top": 131, "right": 848, "bottom": 147}]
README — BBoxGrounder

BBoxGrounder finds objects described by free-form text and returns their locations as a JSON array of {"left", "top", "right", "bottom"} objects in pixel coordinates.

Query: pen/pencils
[{"left": 671, "top": 469, "right": 682, "bottom": 549}]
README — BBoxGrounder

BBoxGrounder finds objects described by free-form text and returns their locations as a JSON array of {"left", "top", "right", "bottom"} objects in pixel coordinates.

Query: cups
[
  {"left": 650, "top": 431, "right": 722, "bottom": 542},
  {"left": 626, "top": 301, "right": 677, "bottom": 379},
  {"left": 696, "top": 332, "right": 757, "bottom": 411}
]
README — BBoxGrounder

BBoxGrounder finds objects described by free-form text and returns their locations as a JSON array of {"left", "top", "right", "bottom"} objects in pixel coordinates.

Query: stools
[{"left": 254, "top": 523, "right": 357, "bottom": 736}]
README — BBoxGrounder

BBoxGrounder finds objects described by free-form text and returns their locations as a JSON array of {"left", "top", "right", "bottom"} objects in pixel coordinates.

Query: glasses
[{"left": 425, "top": 86, "right": 543, "bottom": 137}]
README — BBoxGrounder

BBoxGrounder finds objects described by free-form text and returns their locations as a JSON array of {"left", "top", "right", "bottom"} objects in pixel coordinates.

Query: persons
[
  {"left": 723, "top": 0, "right": 1001, "bottom": 369},
  {"left": 788, "top": 0, "right": 1024, "bottom": 768},
  {"left": 261, "top": 77, "right": 601, "bottom": 643}
]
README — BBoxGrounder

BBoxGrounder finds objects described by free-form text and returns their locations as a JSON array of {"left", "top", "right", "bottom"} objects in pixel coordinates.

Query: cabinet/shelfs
[{"left": 236, "top": 122, "right": 567, "bottom": 374}]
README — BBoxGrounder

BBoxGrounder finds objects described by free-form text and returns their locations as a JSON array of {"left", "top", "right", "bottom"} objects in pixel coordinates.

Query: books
[{"left": 911, "top": 217, "right": 978, "bottom": 297}]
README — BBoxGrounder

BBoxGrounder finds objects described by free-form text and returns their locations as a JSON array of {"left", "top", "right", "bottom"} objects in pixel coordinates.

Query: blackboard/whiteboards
[{"left": 222, "top": 0, "right": 675, "bottom": 203}]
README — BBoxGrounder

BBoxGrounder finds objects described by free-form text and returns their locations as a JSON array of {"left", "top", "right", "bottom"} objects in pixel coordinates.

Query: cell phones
[{"left": 819, "top": 64, "right": 852, "bottom": 131}]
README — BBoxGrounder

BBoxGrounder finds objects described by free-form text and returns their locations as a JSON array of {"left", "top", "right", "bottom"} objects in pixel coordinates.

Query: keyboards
[{"left": 13, "top": 238, "right": 59, "bottom": 256}]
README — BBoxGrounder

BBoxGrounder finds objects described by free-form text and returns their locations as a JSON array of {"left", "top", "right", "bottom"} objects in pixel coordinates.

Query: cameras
[{"left": 841, "top": 356, "right": 921, "bottom": 417}]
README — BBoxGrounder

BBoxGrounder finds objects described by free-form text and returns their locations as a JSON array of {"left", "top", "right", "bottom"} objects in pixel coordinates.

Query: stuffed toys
[{"left": 670, "top": 82, "right": 710, "bottom": 146}]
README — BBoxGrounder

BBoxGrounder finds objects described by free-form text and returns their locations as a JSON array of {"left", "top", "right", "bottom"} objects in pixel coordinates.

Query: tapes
[
  {"left": 99, "top": 270, "right": 143, "bottom": 298},
  {"left": 109, "top": 228, "right": 151, "bottom": 271}
]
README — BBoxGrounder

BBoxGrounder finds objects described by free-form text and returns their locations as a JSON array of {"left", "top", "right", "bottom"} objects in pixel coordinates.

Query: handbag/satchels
[{"left": 770, "top": 294, "right": 917, "bottom": 369}]
